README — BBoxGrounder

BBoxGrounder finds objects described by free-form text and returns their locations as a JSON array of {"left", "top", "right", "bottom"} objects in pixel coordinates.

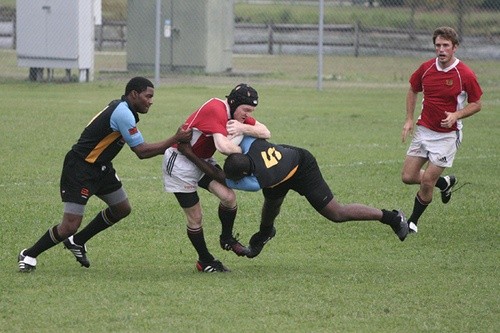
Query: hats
[{"left": 230, "top": 83, "right": 259, "bottom": 107}]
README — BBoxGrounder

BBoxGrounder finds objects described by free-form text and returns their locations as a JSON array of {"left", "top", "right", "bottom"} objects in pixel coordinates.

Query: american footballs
[{"left": 225, "top": 120, "right": 244, "bottom": 146}]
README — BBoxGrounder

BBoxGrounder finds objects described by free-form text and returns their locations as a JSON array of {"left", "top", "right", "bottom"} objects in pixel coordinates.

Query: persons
[
  {"left": 177, "top": 136, "right": 410, "bottom": 258},
  {"left": 18, "top": 76, "right": 193, "bottom": 273},
  {"left": 400, "top": 26, "right": 481, "bottom": 233},
  {"left": 162, "top": 83, "right": 272, "bottom": 274}
]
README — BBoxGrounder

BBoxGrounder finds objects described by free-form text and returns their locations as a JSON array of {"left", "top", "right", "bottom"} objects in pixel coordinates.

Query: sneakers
[
  {"left": 17, "top": 249, "right": 37, "bottom": 274},
  {"left": 63, "top": 235, "right": 90, "bottom": 268},
  {"left": 406, "top": 219, "right": 418, "bottom": 234},
  {"left": 245, "top": 226, "right": 276, "bottom": 258},
  {"left": 390, "top": 209, "right": 408, "bottom": 241},
  {"left": 219, "top": 233, "right": 247, "bottom": 257},
  {"left": 196, "top": 259, "right": 230, "bottom": 273},
  {"left": 440, "top": 175, "right": 456, "bottom": 204}
]
